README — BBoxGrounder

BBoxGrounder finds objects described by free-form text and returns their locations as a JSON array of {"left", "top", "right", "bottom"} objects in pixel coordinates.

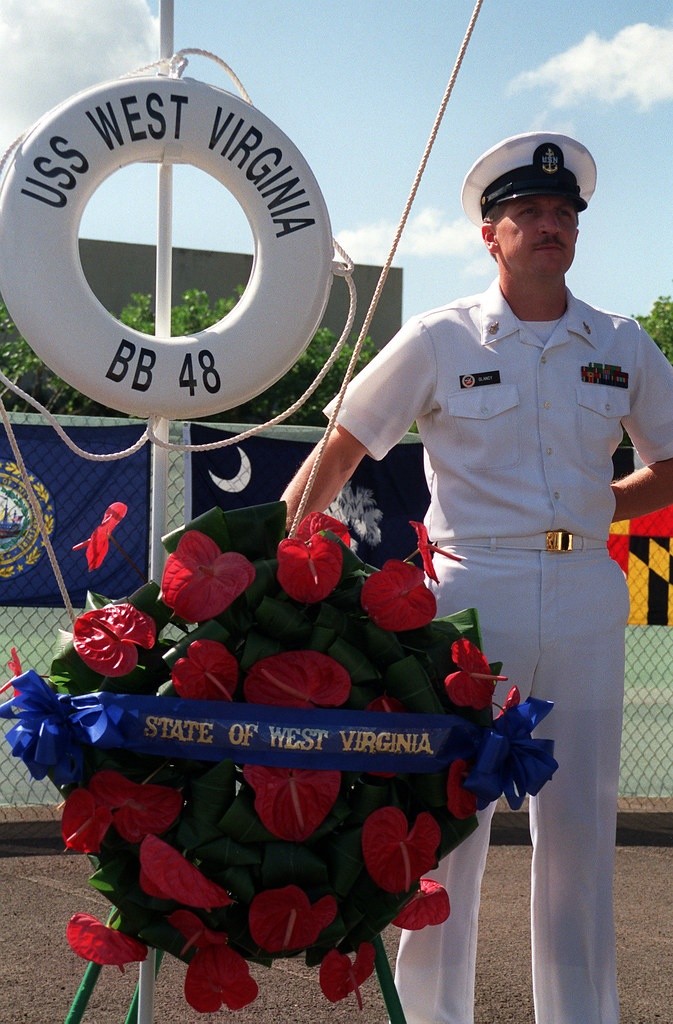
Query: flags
[
  {"left": 184, "top": 424, "right": 431, "bottom": 570},
  {"left": 613, "top": 446, "right": 673, "bottom": 625},
  {"left": 0, "top": 423, "right": 152, "bottom": 609}
]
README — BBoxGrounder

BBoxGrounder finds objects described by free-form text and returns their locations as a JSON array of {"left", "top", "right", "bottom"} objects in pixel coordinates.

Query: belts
[{"left": 437, "top": 529, "right": 608, "bottom": 551}]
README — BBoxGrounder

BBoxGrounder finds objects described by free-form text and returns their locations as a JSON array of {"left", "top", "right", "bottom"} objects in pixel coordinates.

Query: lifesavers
[{"left": 0, "top": 73, "right": 336, "bottom": 422}]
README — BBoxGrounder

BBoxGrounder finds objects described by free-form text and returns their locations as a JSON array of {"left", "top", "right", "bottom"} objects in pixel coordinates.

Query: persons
[{"left": 280, "top": 133, "right": 672, "bottom": 1024}]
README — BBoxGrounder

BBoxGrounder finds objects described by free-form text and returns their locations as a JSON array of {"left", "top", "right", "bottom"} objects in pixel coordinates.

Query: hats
[{"left": 461, "top": 132, "right": 598, "bottom": 227}]
